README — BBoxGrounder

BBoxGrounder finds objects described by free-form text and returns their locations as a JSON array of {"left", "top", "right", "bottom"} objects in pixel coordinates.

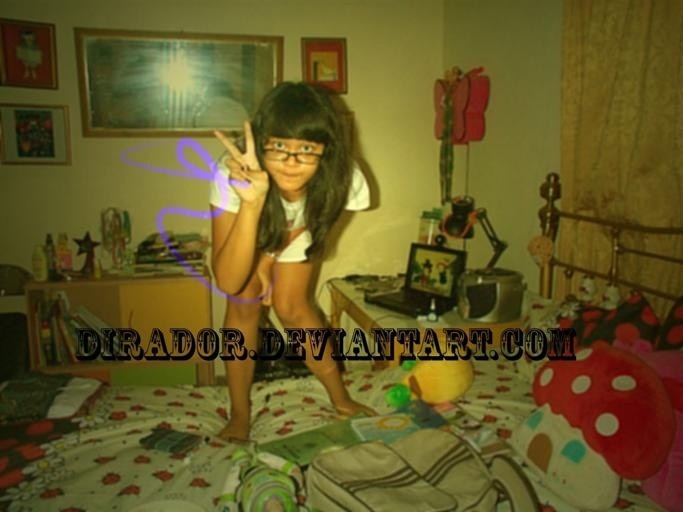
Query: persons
[
  {"left": 209, "top": 80, "right": 379, "bottom": 444},
  {"left": 385, "top": 339, "right": 475, "bottom": 408}
]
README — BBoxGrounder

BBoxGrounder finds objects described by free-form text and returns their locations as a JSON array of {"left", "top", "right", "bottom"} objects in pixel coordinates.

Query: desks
[
  {"left": 325, "top": 275, "right": 529, "bottom": 371},
  {"left": 22, "top": 274, "right": 216, "bottom": 388}
]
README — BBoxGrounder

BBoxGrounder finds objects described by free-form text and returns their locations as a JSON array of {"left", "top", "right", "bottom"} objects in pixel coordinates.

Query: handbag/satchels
[{"left": 305, "top": 429, "right": 499, "bottom": 512}]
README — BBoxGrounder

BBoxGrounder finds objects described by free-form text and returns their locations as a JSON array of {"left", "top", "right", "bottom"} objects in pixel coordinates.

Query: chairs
[{"left": 0, "top": 263, "right": 34, "bottom": 383}]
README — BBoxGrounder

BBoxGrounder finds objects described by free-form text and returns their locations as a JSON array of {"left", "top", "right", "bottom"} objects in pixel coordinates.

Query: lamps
[{"left": 438, "top": 193, "right": 509, "bottom": 267}]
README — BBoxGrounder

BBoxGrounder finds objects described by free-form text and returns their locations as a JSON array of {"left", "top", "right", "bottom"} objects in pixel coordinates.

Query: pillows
[{"left": 505, "top": 288, "right": 683, "bottom": 511}]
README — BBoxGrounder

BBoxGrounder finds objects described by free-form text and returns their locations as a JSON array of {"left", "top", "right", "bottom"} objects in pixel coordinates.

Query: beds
[{"left": 0, "top": 172, "right": 683, "bottom": 512}]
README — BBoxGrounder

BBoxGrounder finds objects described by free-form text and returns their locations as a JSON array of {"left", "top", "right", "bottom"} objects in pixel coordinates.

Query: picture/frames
[
  {"left": 301, "top": 37, "right": 348, "bottom": 94},
  {"left": 0, "top": 102, "right": 72, "bottom": 165},
  {"left": 73, "top": 26, "right": 283, "bottom": 138},
  {"left": 0, "top": 17, "right": 58, "bottom": 90}
]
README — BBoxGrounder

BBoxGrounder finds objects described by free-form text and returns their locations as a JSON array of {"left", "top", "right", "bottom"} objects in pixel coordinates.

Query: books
[
  {"left": 133, "top": 232, "right": 207, "bottom": 276},
  {"left": 36, "top": 295, "right": 129, "bottom": 364}
]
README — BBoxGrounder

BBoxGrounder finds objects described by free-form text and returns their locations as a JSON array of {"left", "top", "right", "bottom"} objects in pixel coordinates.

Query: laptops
[{"left": 364, "top": 242, "right": 468, "bottom": 321}]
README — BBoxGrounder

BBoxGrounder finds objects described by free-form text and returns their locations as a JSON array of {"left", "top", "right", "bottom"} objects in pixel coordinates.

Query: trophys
[{"left": 72, "top": 229, "right": 100, "bottom": 274}]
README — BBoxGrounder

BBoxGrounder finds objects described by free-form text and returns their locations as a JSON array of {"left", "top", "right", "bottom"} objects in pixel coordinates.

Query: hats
[
  {"left": 423, "top": 259, "right": 433, "bottom": 268},
  {"left": 437, "top": 260, "right": 448, "bottom": 268}
]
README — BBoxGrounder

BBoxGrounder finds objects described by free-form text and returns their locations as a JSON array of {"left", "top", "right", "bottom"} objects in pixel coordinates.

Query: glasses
[{"left": 259, "top": 139, "right": 326, "bottom": 164}]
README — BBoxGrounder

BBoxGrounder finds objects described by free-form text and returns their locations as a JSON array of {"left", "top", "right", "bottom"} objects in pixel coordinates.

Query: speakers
[{"left": 456, "top": 266, "right": 527, "bottom": 322}]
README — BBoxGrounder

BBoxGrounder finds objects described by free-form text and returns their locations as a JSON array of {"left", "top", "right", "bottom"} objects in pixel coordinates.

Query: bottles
[{"left": 32, "top": 230, "right": 71, "bottom": 282}]
